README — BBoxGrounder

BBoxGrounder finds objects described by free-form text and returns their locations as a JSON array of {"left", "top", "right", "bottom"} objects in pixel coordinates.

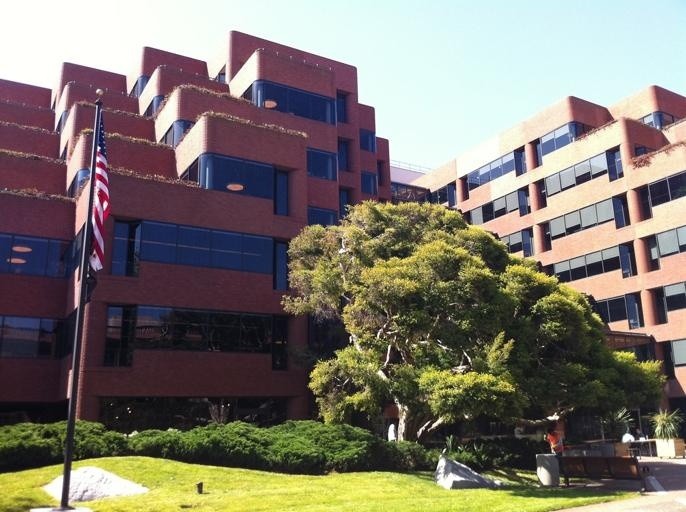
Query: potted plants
[
  {"left": 595, "top": 406, "right": 635, "bottom": 457},
  {"left": 648, "top": 410, "right": 686, "bottom": 460}
]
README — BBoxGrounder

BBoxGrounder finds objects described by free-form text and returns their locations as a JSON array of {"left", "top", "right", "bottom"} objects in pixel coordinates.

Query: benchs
[{"left": 556, "top": 456, "right": 649, "bottom": 492}]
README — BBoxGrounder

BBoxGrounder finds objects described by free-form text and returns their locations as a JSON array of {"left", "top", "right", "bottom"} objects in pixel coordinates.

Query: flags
[{"left": 88, "top": 111, "right": 111, "bottom": 272}]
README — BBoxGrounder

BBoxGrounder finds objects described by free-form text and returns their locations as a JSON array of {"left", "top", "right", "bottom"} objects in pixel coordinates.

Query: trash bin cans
[{"left": 536, "top": 454, "right": 560, "bottom": 486}]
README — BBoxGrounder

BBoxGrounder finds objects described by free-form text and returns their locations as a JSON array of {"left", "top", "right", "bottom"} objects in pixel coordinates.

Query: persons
[
  {"left": 546, "top": 424, "right": 569, "bottom": 487},
  {"left": 621, "top": 428, "right": 646, "bottom": 453}
]
